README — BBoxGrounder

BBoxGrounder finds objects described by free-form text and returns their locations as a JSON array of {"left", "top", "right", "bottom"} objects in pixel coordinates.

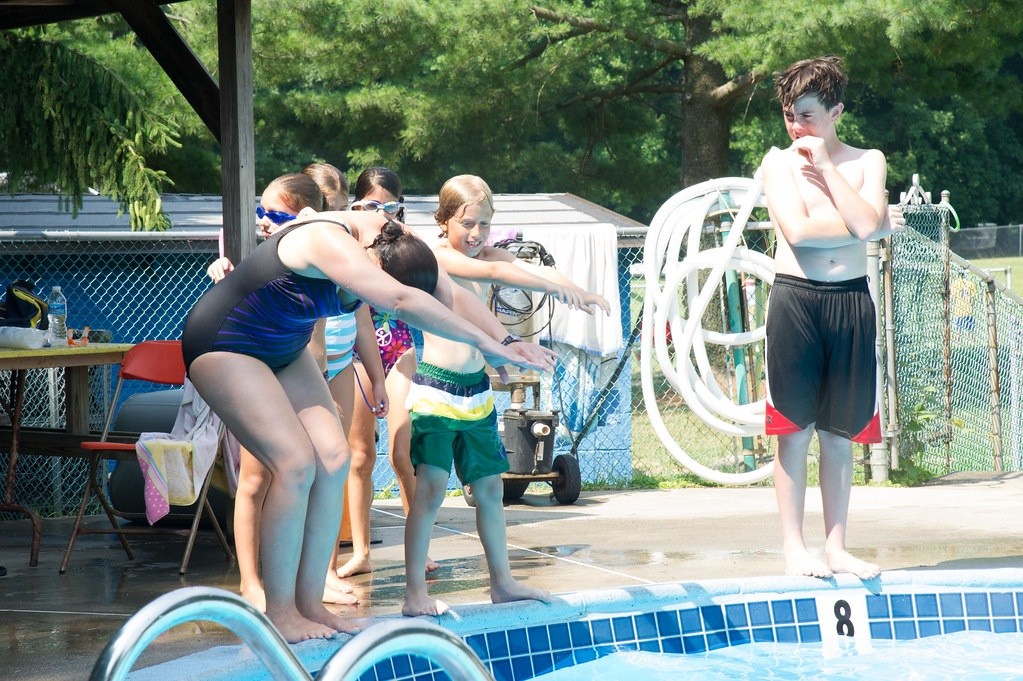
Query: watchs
[{"left": 501, "top": 334, "right": 521, "bottom": 345}]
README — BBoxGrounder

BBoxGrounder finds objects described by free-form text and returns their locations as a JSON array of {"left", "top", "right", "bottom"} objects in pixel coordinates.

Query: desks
[{"left": 0, "top": 340, "right": 136, "bottom": 566}]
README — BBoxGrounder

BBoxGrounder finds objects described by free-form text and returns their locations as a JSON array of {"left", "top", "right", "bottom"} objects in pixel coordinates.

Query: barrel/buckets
[{"left": 503, "top": 406, "right": 559, "bottom": 474}]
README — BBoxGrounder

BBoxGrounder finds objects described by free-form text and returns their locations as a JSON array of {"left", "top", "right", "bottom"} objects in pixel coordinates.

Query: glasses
[
  {"left": 362, "top": 201, "right": 402, "bottom": 214},
  {"left": 68, "top": 327, "right": 90, "bottom": 346},
  {"left": 255, "top": 207, "right": 296, "bottom": 224}
]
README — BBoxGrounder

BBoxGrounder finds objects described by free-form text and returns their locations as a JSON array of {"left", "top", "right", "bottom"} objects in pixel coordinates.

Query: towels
[
  {"left": 135, "top": 372, "right": 242, "bottom": 526},
  {"left": 522, "top": 220, "right": 624, "bottom": 358}
]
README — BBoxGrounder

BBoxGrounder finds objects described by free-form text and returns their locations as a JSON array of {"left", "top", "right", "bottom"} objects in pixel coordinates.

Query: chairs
[{"left": 59, "top": 341, "right": 236, "bottom": 574}]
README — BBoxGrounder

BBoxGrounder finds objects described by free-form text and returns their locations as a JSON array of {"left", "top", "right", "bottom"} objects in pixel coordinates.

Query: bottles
[{"left": 47, "top": 286, "right": 67, "bottom": 348}]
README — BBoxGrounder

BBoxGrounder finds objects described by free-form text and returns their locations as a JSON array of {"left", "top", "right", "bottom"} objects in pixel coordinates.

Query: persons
[
  {"left": 952, "top": 271, "right": 976, "bottom": 341},
  {"left": 403, "top": 173, "right": 610, "bottom": 616},
  {"left": 181, "top": 210, "right": 560, "bottom": 643},
  {"left": 760, "top": 56, "right": 905, "bottom": 578},
  {"left": 207, "top": 162, "right": 438, "bottom": 612}
]
